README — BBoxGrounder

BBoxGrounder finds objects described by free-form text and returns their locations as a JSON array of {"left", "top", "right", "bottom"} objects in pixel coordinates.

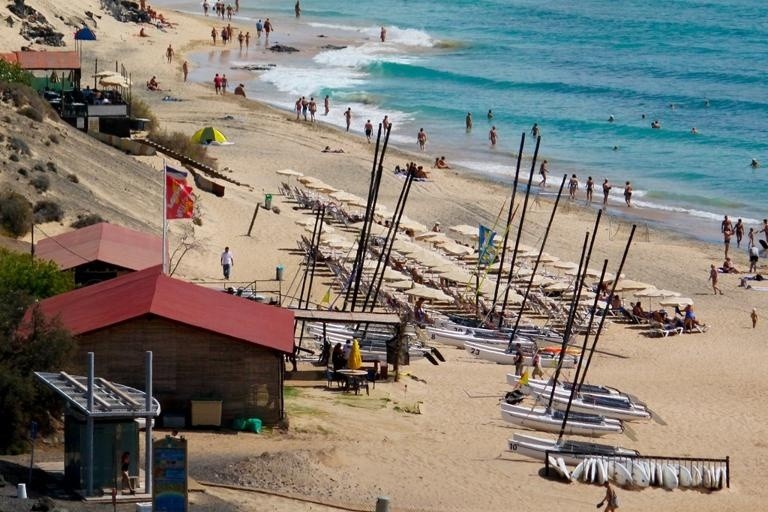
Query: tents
[
  {"left": 74, "top": 28, "right": 96, "bottom": 41},
  {"left": 190, "top": 126, "right": 228, "bottom": 145}
]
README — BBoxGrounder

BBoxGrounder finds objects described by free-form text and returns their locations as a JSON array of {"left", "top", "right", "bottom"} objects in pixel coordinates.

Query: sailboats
[
  {"left": 533, "top": 222, "right": 651, "bottom": 423},
  {"left": 506, "top": 257, "right": 641, "bottom": 467},
  {"left": 462, "top": 173, "right": 579, "bottom": 370},
  {"left": 424, "top": 135, "right": 542, "bottom": 355},
  {"left": 286, "top": 121, "right": 653, "bottom": 479},
  {"left": 512, "top": 223, "right": 632, "bottom": 404},
  {"left": 499, "top": 231, "right": 624, "bottom": 438},
  {"left": 505, "top": 205, "right": 622, "bottom": 397}
]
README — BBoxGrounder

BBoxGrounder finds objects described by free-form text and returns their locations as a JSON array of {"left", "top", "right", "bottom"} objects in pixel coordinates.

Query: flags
[{"left": 163, "top": 164, "right": 195, "bottom": 220}]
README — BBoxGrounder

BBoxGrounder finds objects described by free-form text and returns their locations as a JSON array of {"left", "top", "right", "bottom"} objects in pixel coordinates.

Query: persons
[
  {"left": 332, "top": 343, "right": 344, "bottom": 388},
  {"left": 294, "top": 94, "right": 697, "bottom": 208},
  {"left": 751, "top": 157, "right": 759, "bottom": 168},
  {"left": 751, "top": 308, "right": 759, "bottom": 328},
  {"left": 601, "top": 480, "right": 620, "bottom": 512},
  {"left": 343, "top": 339, "right": 353, "bottom": 360},
  {"left": 532, "top": 349, "right": 545, "bottom": 380},
  {"left": 380, "top": 26, "right": 386, "bottom": 42},
  {"left": 709, "top": 213, "right": 768, "bottom": 295},
  {"left": 139, "top": 0, "right": 274, "bottom": 97},
  {"left": 513, "top": 343, "right": 524, "bottom": 376},
  {"left": 611, "top": 295, "right": 706, "bottom": 334},
  {"left": 295, "top": 0, "right": 302, "bottom": 17},
  {"left": 220, "top": 247, "right": 234, "bottom": 280},
  {"left": 412, "top": 297, "right": 426, "bottom": 322}
]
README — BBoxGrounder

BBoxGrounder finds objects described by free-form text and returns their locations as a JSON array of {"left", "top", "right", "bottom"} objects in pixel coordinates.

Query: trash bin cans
[
  {"left": 265, "top": 194, "right": 272, "bottom": 209},
  {"left": 276, "top": 265, "right": 284, "bottom": 281}
]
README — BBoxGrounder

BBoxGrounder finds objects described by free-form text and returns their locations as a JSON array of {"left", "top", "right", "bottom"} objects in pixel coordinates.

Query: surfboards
[{"left": 758, "top": 239, "right": 768, "bottom": 251}]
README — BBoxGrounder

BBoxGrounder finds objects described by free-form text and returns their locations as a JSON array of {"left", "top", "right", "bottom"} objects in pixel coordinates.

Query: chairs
[{"left": 275, "top": 168, "right": 706, "bottom": 337}]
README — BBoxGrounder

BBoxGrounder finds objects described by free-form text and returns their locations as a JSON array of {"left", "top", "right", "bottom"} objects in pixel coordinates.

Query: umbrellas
[{"left": 348, "top": 339, "right": 363, "bottom": 372}]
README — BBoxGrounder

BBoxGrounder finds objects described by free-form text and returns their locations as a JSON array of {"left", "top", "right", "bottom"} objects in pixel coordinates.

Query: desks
[{"left": 336, "top": 369, "right": 369, "bottom": 397}]
